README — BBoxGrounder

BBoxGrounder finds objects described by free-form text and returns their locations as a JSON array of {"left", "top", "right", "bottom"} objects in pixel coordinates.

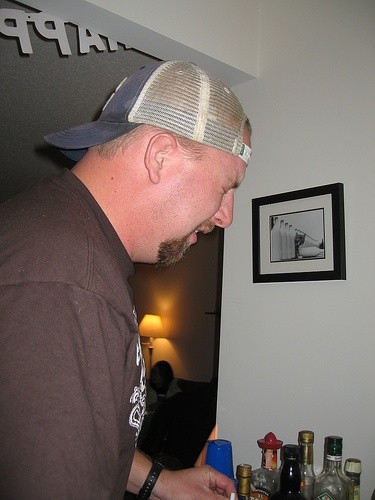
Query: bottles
[
  {"left": 298, "top": 430, "right": 316, "bottom": 500},
  {"left": 312, "top": 435, "right": 354, "bottom": 500},
  {"left": 250, "top": 431, "right": 283, "bottom": 500},
  {"left": 236, "top": 463, "right": 253, "bottom": 500},
  {"left": 270, "top": 216, "right": 324, "bottom": 262},
  {"left": 268, "top": 444, "right": 308, "bottom": 500},
  {"left": 344, "top": 458, "right": 361, "bottom": 500}
]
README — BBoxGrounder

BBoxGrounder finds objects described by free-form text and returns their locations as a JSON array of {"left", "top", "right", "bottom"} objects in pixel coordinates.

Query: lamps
[{"left": 139, "top": 314, "right": 166, "bottom": 373}]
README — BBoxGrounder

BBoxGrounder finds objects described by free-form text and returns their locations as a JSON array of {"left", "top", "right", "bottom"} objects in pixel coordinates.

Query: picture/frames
[{"left": 251, "top": 183, "right": 346, "bottom": 285}]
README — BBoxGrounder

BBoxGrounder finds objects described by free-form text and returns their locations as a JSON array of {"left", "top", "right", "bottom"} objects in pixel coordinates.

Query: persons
[
  {"left": 137, "top": 361, "right": 183, "bottom": 457},
  {"left": 0, "top": 61, "right": 251, "bottom": 500}
]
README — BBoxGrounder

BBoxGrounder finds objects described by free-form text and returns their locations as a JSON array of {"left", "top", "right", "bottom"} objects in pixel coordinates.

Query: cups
[{"left": 205, "top": 438, "right": 238, "bottom": 486}]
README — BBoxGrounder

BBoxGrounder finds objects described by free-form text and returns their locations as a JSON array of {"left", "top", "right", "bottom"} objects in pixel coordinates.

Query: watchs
[{"left": 139, "top": 457, "right": 167, "bottom": 499}]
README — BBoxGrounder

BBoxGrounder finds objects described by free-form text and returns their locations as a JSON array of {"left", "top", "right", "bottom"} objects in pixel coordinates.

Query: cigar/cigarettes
[{"left": 230, "top": 492, "right": 235, "bottom": 500}]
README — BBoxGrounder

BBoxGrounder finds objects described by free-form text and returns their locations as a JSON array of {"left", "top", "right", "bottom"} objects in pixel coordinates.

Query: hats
[{"left": 44, "top": 60, "right": 253, "bottom": 165}]
